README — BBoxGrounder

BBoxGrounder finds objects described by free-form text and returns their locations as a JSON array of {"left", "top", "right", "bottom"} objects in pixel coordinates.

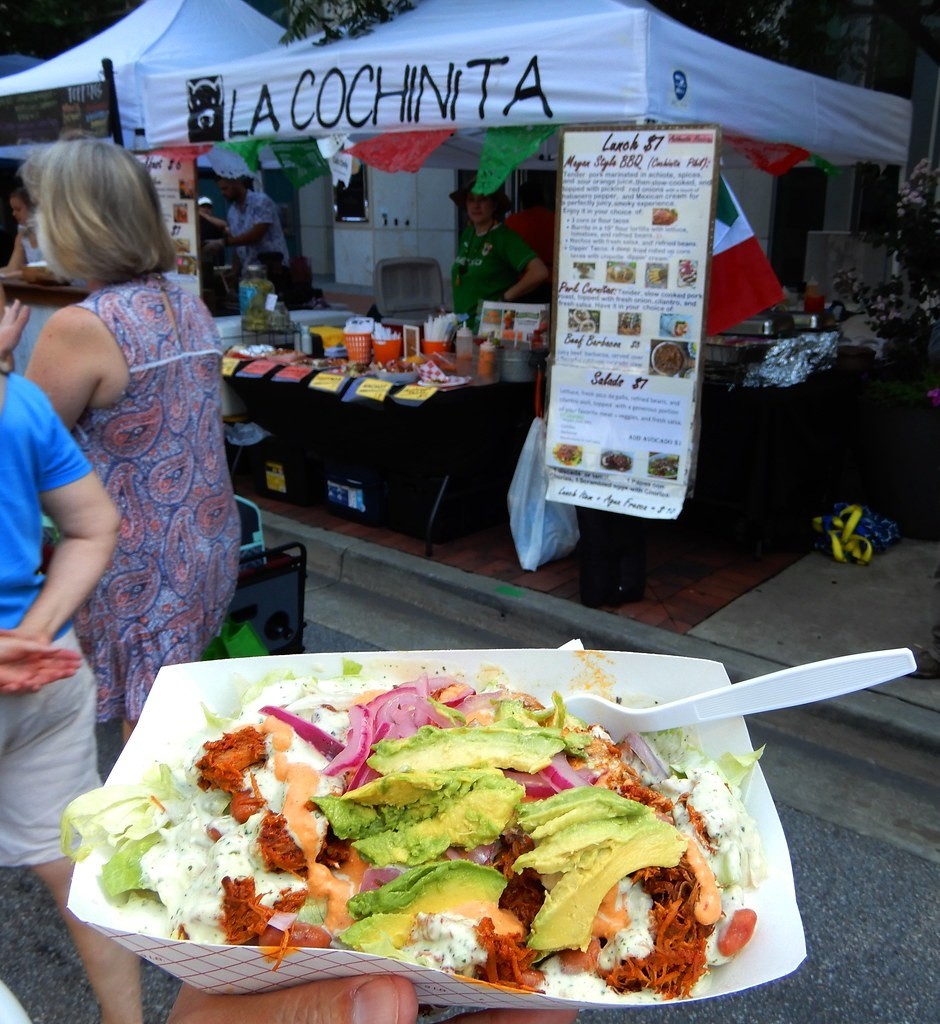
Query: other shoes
[{"left": 906, "top": 650, "right": 940, "bottom": 679}]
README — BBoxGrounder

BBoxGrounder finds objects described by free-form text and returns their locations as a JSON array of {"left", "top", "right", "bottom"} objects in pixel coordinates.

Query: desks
[{"left": 220, "top": 352, "right": 549, "bottom": 559}]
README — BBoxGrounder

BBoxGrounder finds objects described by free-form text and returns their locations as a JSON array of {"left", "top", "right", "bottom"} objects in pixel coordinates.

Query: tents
[{"left": 0, "top": 0, "right": 913, "bottom": 319}]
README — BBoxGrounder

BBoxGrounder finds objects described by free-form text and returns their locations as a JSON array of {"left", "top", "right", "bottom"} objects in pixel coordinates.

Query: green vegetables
[
  {"left": 61, "top": 704, "right": 238, "bottom": 904},
  {"left": 644, "top": 727, "right": 767, "bottom": 887}
]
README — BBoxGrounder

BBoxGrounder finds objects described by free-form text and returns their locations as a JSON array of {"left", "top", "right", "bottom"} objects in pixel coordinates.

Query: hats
[{"left": 449, "top": 176, "right": 513, "bottom": 215}]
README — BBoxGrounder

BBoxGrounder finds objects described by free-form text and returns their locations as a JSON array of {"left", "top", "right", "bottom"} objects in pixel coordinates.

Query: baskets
[{"left": 344, "top": 332, "right": 372, "bottom": 366}]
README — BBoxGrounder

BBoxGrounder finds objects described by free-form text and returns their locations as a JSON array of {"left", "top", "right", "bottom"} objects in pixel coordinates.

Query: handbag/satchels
[
  {"left": 506, "top": 367, "right": 581, "bottom": 574},
  {"left": 811, "top": 501, "right": 900, "bottom": 566},
  {"left": 203, "top": 620, "right": 268, "bottom": 660}
]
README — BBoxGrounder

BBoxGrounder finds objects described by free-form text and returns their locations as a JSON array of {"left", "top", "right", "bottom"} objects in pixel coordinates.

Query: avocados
[{"left": 311, "top": 692, "right": 690, "bottom": 966}]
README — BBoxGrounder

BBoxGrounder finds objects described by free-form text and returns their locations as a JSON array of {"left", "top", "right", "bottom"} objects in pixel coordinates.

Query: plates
[
  {"left": 644, "top": 264, "right": 665, "bottom": 285},
  {"left": 374, "top": 371, "right": 418, "bottom": 384},
  {"left": 416, "top": 376, "right": 466, "bottom": 387},
  {"left": 569, "top": 308, "right": 596, "bottom": 332},
  {"left": 607, "top": 265, "right": 636, "bottom": 284},
  {"left": 573, "top": 266, "right": 594, "bottom": 280}
]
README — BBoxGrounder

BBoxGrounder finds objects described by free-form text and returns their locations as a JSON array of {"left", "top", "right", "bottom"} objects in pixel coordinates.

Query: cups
[
  {"left": 789, "top": 292, "right": 798, "bottom": 310},
  {"left": 422, "top": 339, "right": 451, "bottom": 356},
  {"left": 372, "top": 339, "right": 401, "bottom": 362}
]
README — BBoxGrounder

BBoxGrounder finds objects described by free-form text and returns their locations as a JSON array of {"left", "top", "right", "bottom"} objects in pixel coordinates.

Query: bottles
[
  {"left": 477, "top": 336, "right": 495, "bottom": 375},
  {"left": 238, "top": 266, "right": 289, "bottom": 331},
  {"left": 777, "top": 286, "right": 789, "bottom": 312},
  {"left": 798, "top": 295, "right": 804, "bottom": 311},
  {"left": 455, "top": 320, "right": 474, "bottom": 374},
  {"left": 811, "top": 313, "right": 817, "bottom": 327},
  {"left": 651, "top": 341, "right": 683, "bottom": 374},
  {"left": 805, "top": 277, "right": 820, "bottom": 298}
]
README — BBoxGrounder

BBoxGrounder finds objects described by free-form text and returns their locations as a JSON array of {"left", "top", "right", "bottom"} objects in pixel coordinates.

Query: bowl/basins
[{"left": 497, "top": 346, "right": 549, "bottom": 383}]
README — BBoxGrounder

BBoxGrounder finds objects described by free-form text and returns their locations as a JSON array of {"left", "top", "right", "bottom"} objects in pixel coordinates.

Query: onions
[{"left": 258, "top": 674, "right": 670, "bottom": 895}]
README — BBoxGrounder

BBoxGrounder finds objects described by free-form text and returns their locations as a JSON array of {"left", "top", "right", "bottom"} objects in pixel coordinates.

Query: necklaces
[{"left": 464, "top": 218, "right": 494, "bottom": 272}]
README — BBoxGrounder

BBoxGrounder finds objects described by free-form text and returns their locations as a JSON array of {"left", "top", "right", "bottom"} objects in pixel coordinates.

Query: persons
[
  {"left": 197, "top": 196, "right": 227, "bottom": 294},
  {"left": 202, "top": 175, "right": 294, "bottom": 298},
  {"left": 449, "top": 173, "right": 549, "bottom": 339},
  {"left": 0, "top": 131, "right": 241, "bottom": 1024},
  {"left": 504, "top": 182, "right": 555, "bottom": 304}
]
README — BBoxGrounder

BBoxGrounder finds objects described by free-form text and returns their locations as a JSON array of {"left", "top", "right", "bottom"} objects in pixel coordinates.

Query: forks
[{"left": 562, "top": 647, "right": 918, "bottom": 746}]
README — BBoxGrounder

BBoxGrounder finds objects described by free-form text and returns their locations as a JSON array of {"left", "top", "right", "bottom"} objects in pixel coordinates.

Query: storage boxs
[{"left": 64, "top": 647, "right": 809, "bottom": 1010}]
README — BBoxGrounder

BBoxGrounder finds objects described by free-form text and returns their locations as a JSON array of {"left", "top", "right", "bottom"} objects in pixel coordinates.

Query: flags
[{"left": 701, "top": 171, "right": 784, "bottom": 338}]
